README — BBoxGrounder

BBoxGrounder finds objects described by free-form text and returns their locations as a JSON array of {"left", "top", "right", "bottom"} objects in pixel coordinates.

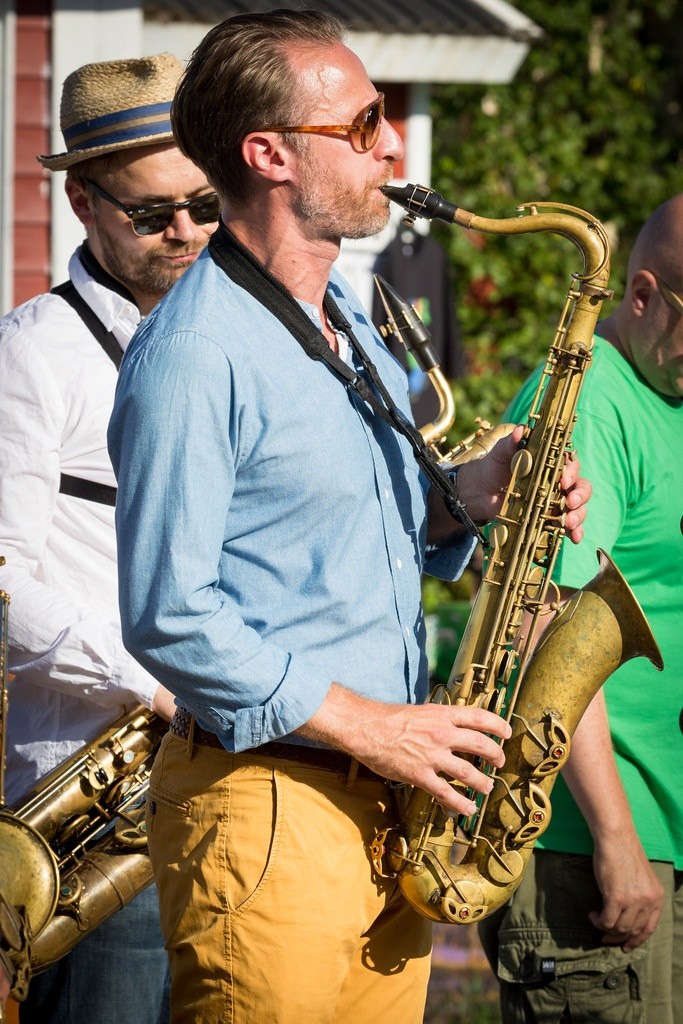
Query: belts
[{"left": 169, "top": 707, "right": 384, "bottom": 780}]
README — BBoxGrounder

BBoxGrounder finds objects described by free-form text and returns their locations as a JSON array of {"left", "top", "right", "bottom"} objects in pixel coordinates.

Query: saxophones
[
  {"left": 0, "top": 700, "right": 162, "bottom": 1002},
  {"left": 370, "top": 177, "right": 669, "bottom": 931}
]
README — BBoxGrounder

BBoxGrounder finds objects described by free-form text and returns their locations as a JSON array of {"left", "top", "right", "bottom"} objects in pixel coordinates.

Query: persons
[
  {"left": 0, "top": 52, "right": 219, "bottom": 1024},
  {"left": 478, "top": 193, "right": 683, "bottom": 1024},
  {"left": 108, "top": 9, "right": 591, "bottom": 1024}
]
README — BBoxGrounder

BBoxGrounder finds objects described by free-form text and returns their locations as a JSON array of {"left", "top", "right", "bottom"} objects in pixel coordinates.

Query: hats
[{"left": 36, "top": 50, "right": 187, "bottom": 173}]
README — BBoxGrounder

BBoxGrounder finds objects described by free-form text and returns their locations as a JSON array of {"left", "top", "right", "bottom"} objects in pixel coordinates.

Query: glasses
[
  {"left": 237, "top": 92, "right": 385, "bottom": 152},
  {"left": 74, "top": 175, "right": 222, "bottom": 236}
]
